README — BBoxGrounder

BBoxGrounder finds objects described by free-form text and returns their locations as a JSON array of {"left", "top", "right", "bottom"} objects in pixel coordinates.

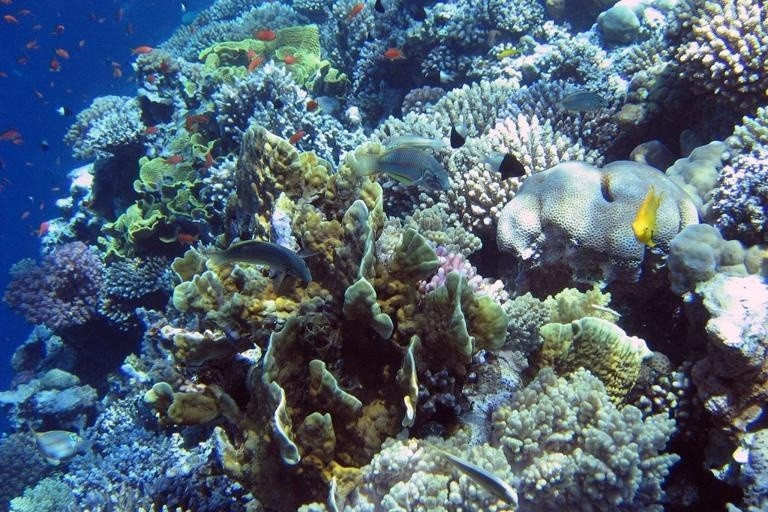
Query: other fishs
[{"left": 0, "top": 0, "right": 566, "bottom": 246}]
